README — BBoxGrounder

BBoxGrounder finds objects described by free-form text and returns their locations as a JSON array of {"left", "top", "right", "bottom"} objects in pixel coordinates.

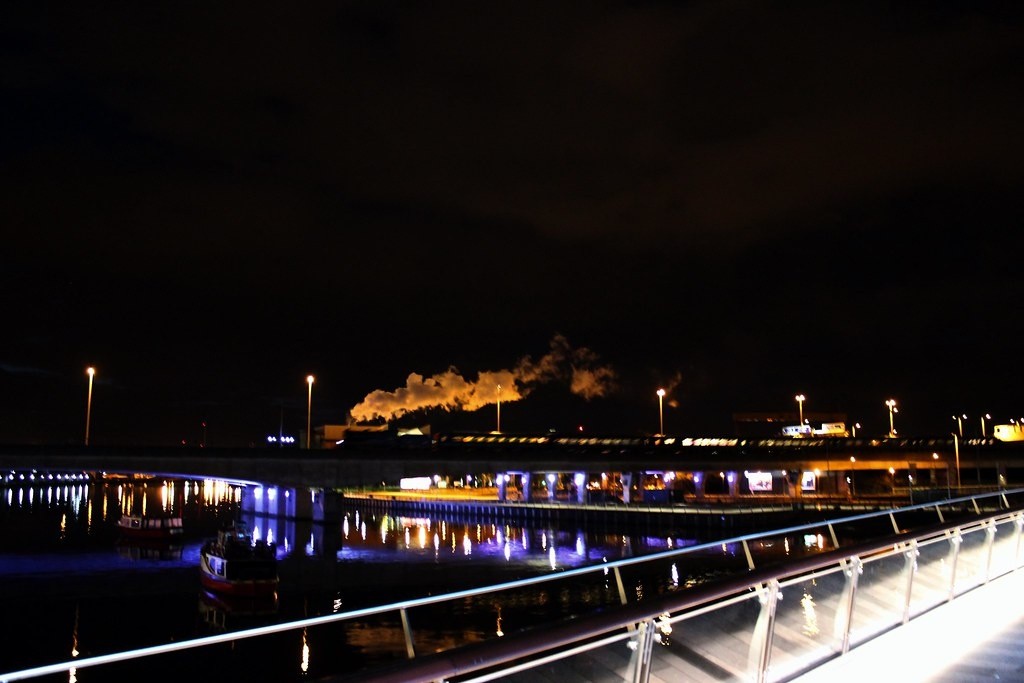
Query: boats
[
  {"left": 118, "top": 507, "right": 187, "bottom": 540},
  {"left": 198, "top": 509, "right": 284, "bottom": 600}
]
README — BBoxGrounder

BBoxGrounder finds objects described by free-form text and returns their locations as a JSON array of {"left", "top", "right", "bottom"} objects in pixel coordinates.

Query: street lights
[
  {"left": 86, "top": 369, "right": 95, "bottom": 445},
  {"left": 795, "top": 395, "right": 806, "bottom": 426},
  {"left": 852, "top": 423, "right": 860, "bottom": 439},
  {"left": 887, "top": 400, "right": 895, "bottom": 435},
  {"left": 656, "top": 388, "right": 665, "bottom": 435},
  {"left": 981, "top": 414, "right": 990, "bottom": 437},
  {"left": 952, "top": 415, "right": 967, "bottom": 436},
  {"left": 307, "top": 374, "right": 314, "bottom": 450}
]
